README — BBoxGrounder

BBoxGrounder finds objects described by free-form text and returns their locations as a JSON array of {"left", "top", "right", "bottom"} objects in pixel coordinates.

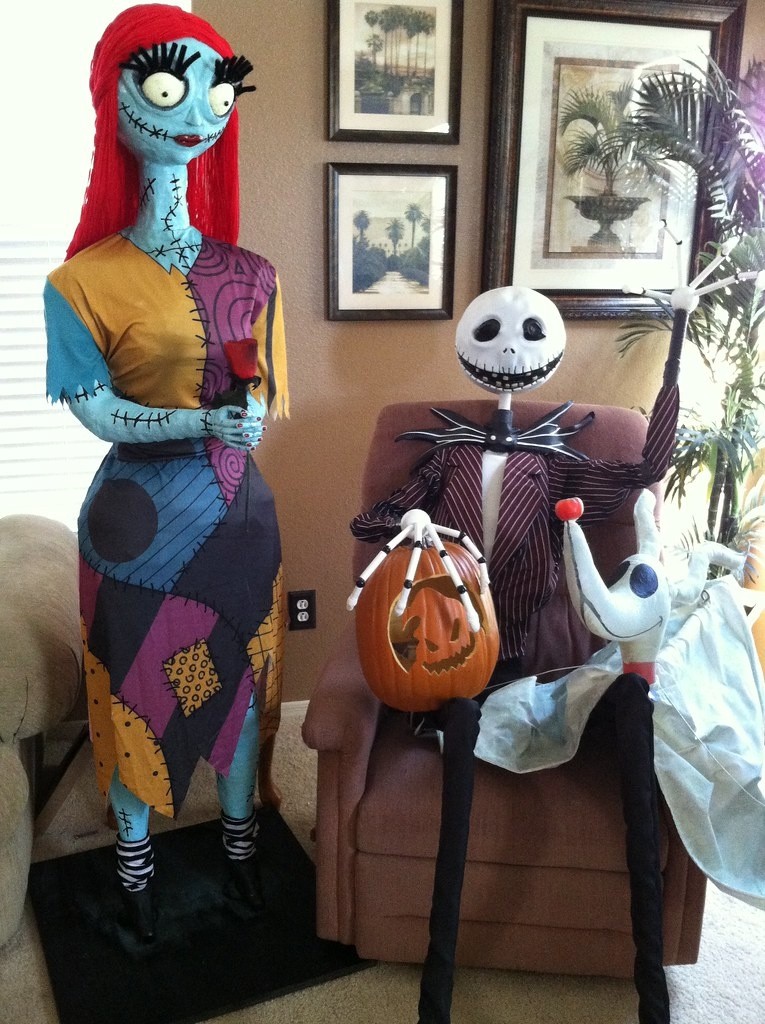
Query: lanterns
[{"left": 353, "top": 537, "right": 500, "bottom": 712}]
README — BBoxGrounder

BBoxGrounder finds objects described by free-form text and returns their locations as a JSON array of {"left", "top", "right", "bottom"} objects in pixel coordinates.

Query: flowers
[{"left": 225, "top": 338, "right": 262, "bottom": 531}]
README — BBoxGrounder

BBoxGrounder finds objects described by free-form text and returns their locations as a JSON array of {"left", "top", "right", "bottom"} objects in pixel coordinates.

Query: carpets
[{"left": 28, "top": 804, "right": 378, "bottom": 1024}]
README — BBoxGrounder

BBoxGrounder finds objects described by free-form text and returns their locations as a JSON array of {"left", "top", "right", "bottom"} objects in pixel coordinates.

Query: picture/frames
[
  {"left": 481, "top": 0, "right": 746, "bottom": 322},
  {"left": 325, "top": 162, "right": 454, "bottom": 321},
  {"left": 326, "top": 0, "right": 463, "bottom": 145}
]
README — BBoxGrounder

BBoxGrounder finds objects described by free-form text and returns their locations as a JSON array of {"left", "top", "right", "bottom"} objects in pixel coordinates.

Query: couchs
[{"left": 300, "top": 398, "right": 705, "bottom": 979}]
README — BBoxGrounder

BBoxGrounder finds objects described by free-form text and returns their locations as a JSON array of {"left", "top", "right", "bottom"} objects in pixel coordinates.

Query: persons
[
  {"left": 44, "top": 4, "right": 288, "bottom": 942},
  {"left": 351, "top": 220, "right": 757, "bottom": 1024}
]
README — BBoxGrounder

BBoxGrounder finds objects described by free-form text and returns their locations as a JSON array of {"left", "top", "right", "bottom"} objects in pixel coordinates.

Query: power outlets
[{"left": 288, "top": 590, "right": 317, "bottom": 632}]
